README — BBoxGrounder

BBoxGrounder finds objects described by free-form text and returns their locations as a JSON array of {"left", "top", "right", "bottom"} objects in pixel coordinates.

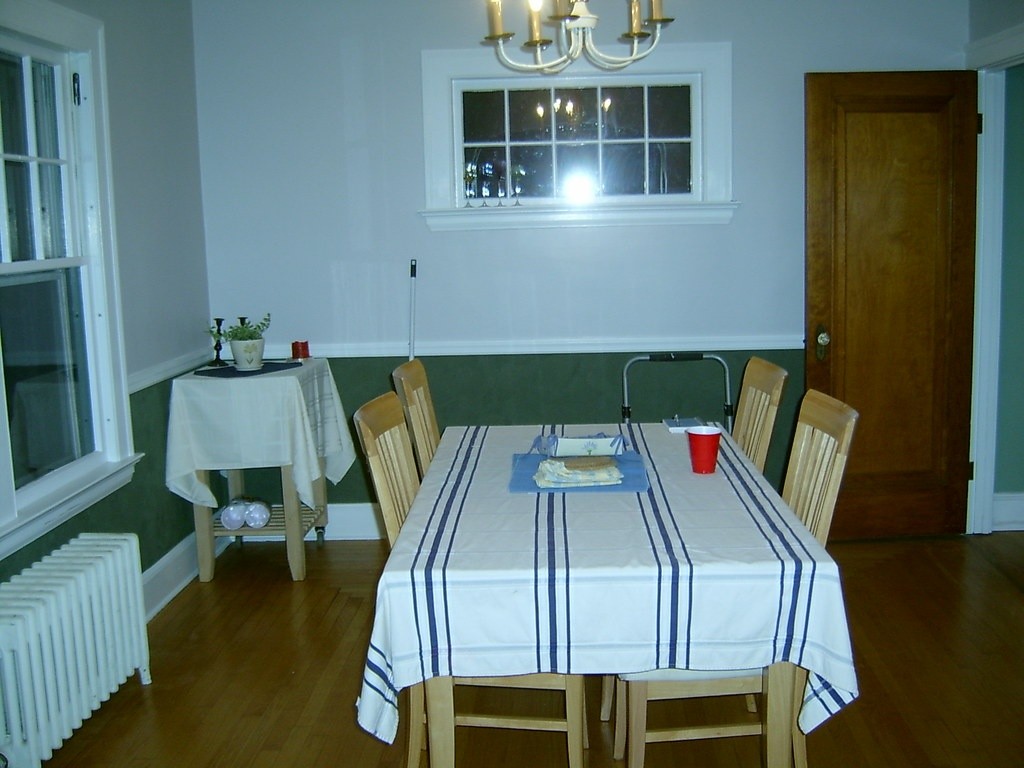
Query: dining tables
[{"left": 355, "top": 422, "right": 860, "bottom": 768}]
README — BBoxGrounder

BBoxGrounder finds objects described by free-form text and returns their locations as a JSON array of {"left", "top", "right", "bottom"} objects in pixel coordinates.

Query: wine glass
[{"left": 460, "top": 160, "right": 528, "bottom": 208}]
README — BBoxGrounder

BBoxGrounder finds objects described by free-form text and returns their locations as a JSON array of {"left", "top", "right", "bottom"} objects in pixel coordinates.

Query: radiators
[{"left": 0, "top": 533, "right": 152, "bottom": 768}]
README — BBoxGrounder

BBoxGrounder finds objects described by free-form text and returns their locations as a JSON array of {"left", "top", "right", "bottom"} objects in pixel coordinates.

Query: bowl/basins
[{"left": 220, "top": 497, "right": 271, "bottom": 530}]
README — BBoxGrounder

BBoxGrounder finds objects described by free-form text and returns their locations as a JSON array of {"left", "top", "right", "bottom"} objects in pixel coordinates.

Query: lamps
[{"left": 483, "top": 0, "right": 675, "bottom": 75}]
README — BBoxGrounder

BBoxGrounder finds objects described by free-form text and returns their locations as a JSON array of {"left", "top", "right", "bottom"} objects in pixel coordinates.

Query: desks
[
  {"left": 12, "top": 366, "right": 96, "bottom": 472},
  {"left": 165, "top": 359, "right": 357, "bottom": 582}
]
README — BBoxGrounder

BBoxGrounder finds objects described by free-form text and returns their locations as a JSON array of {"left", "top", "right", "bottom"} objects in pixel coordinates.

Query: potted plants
[{"left": 204, "top": 313, "right": 271, "bottom": 371}]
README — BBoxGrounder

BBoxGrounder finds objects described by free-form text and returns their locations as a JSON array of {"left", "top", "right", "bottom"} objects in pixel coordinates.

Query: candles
[{"left": 291, "top": 340, "right": 308, "bottom": 359}]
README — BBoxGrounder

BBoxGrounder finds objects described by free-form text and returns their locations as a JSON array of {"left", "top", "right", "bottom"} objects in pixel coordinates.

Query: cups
[{"left": 684, "top": 425, "right": 722, "bottom": 475}]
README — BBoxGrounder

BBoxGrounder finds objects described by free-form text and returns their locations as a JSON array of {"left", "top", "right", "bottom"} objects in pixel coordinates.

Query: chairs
[
  {"left": 392, "top": 358, "right": 440, "bottom": 481},
  {"left": 353, "top": 391, "right": 589, "bottom": 768},
  {"left": 613, "top": 390, "right": 860, "bottom": 768},
  {"left": 599, "top": 357, "right": 789, "bottom": 722}
]
